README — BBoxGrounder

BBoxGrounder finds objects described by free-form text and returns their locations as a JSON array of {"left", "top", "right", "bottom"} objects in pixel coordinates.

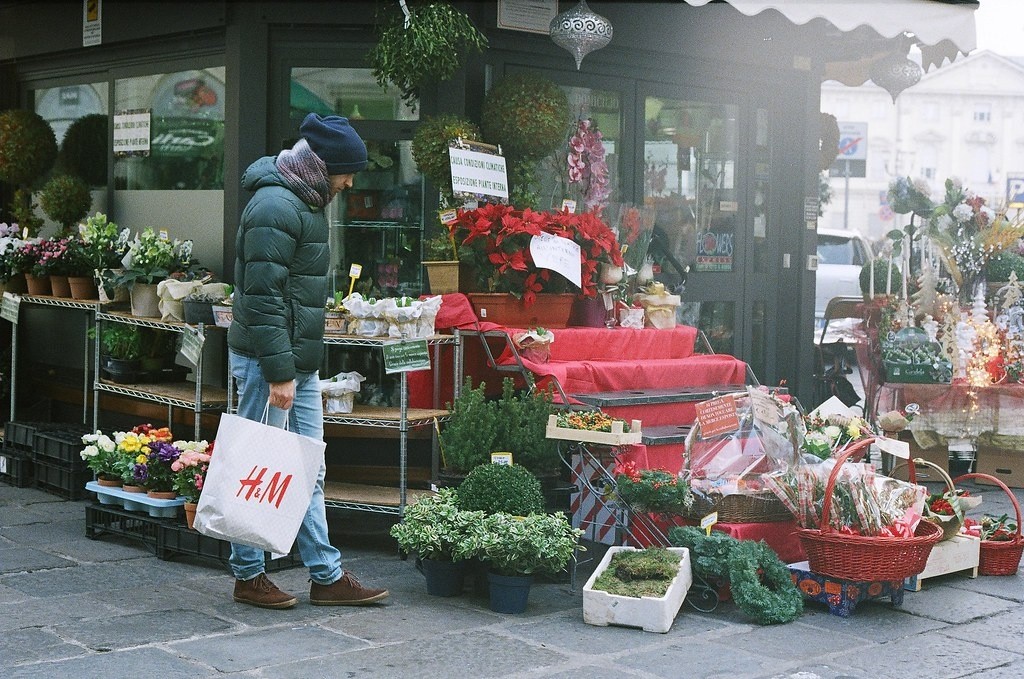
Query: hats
[{"left": 300, "top": 111, "right": 369, "bottom": 175}]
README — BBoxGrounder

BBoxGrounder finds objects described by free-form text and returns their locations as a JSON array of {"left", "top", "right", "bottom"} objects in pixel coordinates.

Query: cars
[{"left": 816, "top": 228, "right": 876, "bottom": 328}]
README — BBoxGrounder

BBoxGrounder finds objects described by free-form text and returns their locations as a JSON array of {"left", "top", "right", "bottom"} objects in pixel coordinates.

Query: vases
[
  {"left": 97, "top": 476, "right": 199, "bottom": 530},
  {"left": 25, "top": 274, "right": 159, "bottom": 319},
  {"left": 466, "top": 291, "right": 574, "bottom": 328}
]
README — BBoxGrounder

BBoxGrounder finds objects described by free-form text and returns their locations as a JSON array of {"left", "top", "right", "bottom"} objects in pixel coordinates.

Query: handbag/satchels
[{"left": 191, "top": 396, "right": 328, "bottom": 555}]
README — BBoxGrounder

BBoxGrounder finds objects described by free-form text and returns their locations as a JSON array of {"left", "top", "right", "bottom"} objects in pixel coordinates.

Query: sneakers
[
  {"left": 309, "top": 570, "right": 391, "bottom": 607},
  {"left": 232, "top": 573, "right": 297, "bottom": 609}
]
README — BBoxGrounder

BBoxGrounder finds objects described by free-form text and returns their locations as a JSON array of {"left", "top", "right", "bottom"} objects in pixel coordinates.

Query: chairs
[{"left": 808, "top": 295, "right": 867, "bottom": 398}]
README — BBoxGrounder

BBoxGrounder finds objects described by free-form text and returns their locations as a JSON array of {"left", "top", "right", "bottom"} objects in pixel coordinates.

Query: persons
[{"left": 229, "top": 112, "right": 390, "bottom": 610}]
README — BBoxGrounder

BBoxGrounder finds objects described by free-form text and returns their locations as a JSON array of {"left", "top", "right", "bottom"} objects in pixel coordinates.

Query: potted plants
[
  {"left": 180, "top": 285, "right": 236, "bottom": 328},
  {"left": 388, "top": 487, "right": 587, "bottom": 616},
  {"left": 86, "top": 322, "right": 142, "bottom": 383},
  {"left": 582, "top": 543, "right": 692, "bottom": 633},
  {"left": 420, "top": 209, "right": 466, "bottom": 295}
]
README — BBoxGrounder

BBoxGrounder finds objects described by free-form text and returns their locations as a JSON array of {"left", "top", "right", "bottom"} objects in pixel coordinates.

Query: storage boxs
[
  {"left": 895, "top": 428, "right": 949, "bottom": 481},
  {"left": 974, "top": 432, "right": 1024, "bottom": 489},
  {"left": 880, "top": 361, "right": 954, "bottom": 384},
  {"left": 0, "top": 419, "right": 109, "bottom": 500},
  {"left": 82, "top": 503, "right": 304, "bottom": 572}
]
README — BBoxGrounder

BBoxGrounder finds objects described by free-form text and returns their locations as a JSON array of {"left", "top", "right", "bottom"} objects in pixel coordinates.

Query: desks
[{"left": 882, "top": 381, "right": 1024, "bottom": 475}]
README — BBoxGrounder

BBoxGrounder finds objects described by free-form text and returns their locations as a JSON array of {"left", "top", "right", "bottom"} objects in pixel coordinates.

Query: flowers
[
  {"left": 453, "top": 206, "right": 625, "bottom": 305},
  {"left": 550, "top": 115, "right": 610, "bottom": 214},
  {"left": 554, "top": 407, "right": 1019, "bottom": 544},
  {"left": 79, "top": 422, "right": 215, "bottom": 503},
  {"left": 0, "top": 211, "right": 193, "bottom": 299}
]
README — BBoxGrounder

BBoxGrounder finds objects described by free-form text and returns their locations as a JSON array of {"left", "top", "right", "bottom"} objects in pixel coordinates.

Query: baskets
[
  {"left": 676, "top": 391, "right": 817, "bottom": 523},
  {"left": 889, "top": 456, "right": 966, "bottom": 543},
  {"left": 791, "top": 439, "right": 944, "bottom": 581},
  {"left": 943, "top": 472, "right": 1024, "bottom": 577}
]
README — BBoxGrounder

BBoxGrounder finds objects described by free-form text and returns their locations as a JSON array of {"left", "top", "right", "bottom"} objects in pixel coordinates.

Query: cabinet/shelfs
[
  {"left": 224, "top": 326, "right": 462, "bottom": 560},
  {"left": 93, "top": 300, "right": 231, "bottom": 438}
]
